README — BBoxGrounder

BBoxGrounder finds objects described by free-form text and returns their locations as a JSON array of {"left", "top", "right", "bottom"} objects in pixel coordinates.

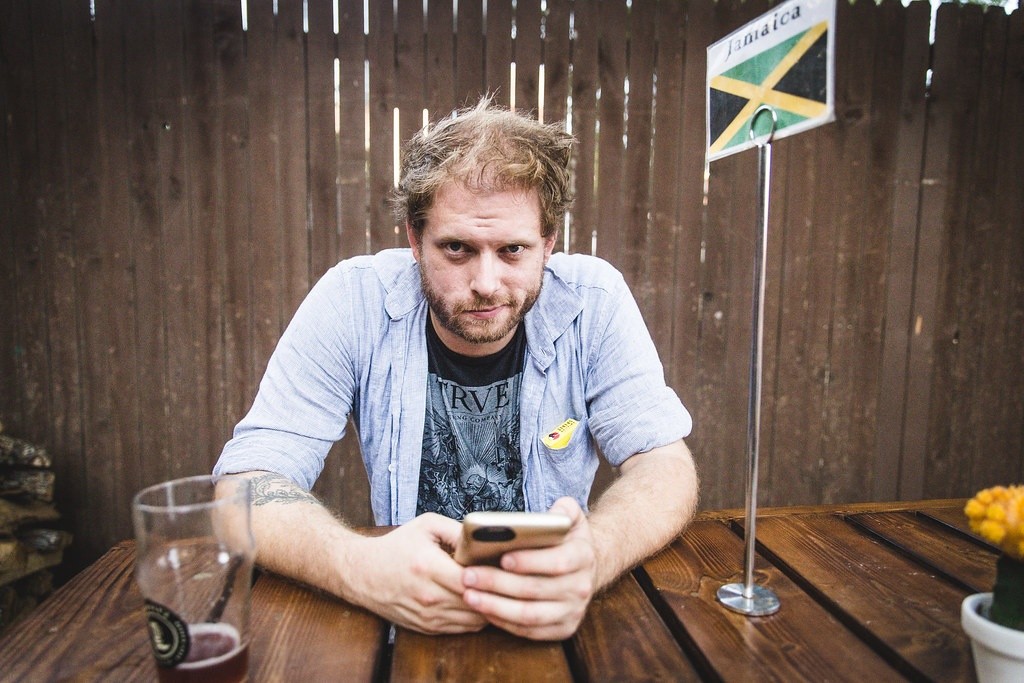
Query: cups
[{"left": 131, "top": 473, "right": 258, "bottom": 671}]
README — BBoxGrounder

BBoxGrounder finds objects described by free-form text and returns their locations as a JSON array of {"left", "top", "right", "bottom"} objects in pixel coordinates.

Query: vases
[{"left": 962, "top": 593, "right": 1024, "bottom": 683}]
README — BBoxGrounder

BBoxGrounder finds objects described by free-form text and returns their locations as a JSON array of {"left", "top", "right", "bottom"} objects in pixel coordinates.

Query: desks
[{"left": 0, "top": 498, "right": 1003, "bottom": 683}]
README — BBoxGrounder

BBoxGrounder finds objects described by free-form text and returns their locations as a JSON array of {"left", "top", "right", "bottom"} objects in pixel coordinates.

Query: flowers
[{"left": 966, "top": 483, "right": 1024, "bottom": 631}]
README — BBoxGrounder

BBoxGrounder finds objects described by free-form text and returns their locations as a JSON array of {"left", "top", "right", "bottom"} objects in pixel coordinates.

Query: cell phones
[{"left": 454, "top": 511, "right": 572, "bottom": 568}]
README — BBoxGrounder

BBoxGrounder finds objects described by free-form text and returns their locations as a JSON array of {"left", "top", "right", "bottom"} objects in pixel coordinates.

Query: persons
[{"left": 208, "top": 105, "right": 700, "bottom": 641}]
